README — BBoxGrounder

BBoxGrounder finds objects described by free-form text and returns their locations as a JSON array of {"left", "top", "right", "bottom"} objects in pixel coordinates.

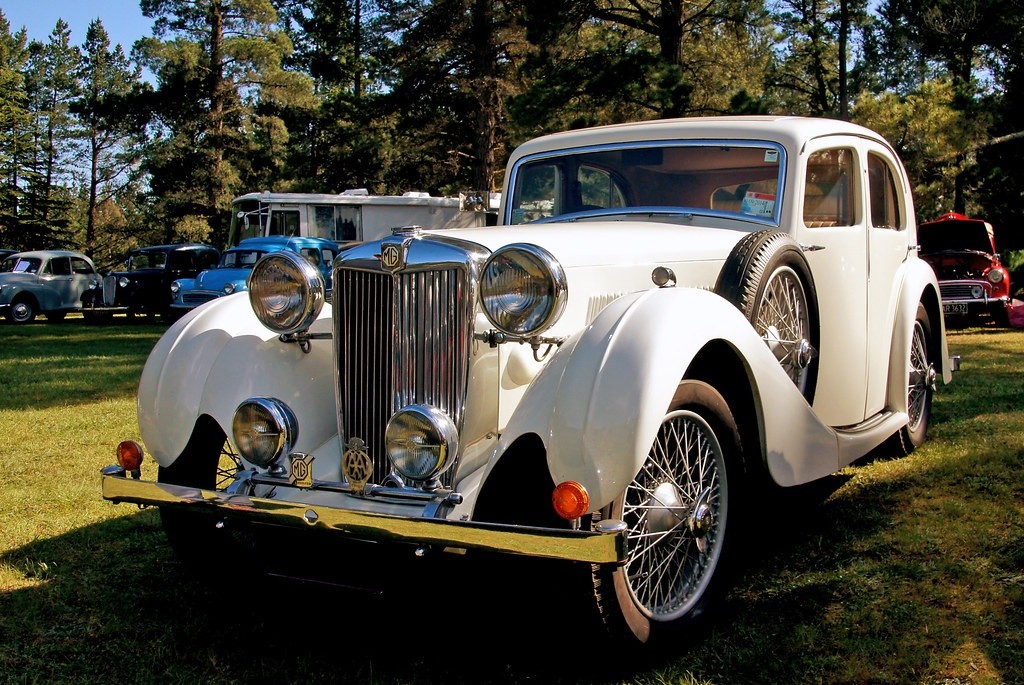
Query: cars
[
  {"left": 80, "top": 243, "right": 220, "bottom": 322},
  {"left": 914, "top": 211, "right": 1011, "bottom": 329},
  {"left": 168, "top": 234, "right": 341, "bottom": 323},
  {"left": 0, "top": 250, "right": 104, "bottom": 324},
  {"left": 100, "top": 114, "right": 963, "bottom": 667},
  {"left": 0, "top": 248, "right": 32, "bottom": 272}
]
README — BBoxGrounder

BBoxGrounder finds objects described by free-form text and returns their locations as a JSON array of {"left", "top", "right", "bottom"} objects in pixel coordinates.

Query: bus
[{"left": 223, "top": 187, "right": 503, "bottom": 247}]
